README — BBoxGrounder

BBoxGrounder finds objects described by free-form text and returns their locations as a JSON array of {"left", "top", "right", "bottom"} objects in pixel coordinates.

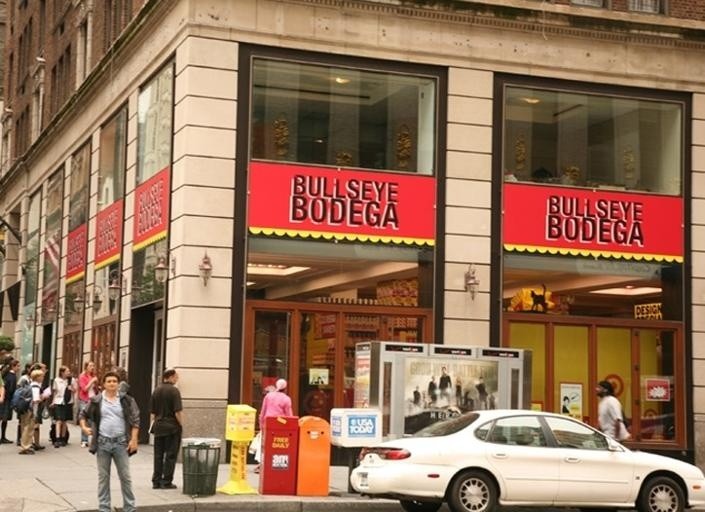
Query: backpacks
[{"left": 11, "top": 385, "right": 40, "bottom": 414}]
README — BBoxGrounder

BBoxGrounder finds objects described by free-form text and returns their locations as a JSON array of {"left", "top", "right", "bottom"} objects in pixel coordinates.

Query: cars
[{"left": 349, "top": 408, "right": 705, "bottom": 512}]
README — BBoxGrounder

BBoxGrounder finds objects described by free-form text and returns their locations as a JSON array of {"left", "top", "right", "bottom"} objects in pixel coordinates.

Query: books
[{"left": 147, "top": 420, "right": 155, "bottom": 433}]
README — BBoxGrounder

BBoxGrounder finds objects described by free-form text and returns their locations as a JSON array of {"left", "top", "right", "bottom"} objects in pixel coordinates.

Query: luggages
[{"left": 50, "top": 415, "right": 69, "bottom": 445}]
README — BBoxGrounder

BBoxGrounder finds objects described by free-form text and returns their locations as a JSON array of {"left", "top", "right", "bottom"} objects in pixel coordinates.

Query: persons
[
  {"left": 0, "top": 354, "right": 131, "bottom": 455},
  {"left": 563, "top": 395, "right": 570, "bottom": 412},
  {"left": 77, "top": 372, "right": 141, "bottom": 511},
  {"left": 594, "top": 381, "right": 633, "bottom": 443},
  {"left": 253, "top": 377, "right": 293, "bottom": 474},
  {"left": 147, "top": 367, "right": 184, "bottom": 489},
  {"left": 412, "top": 366, "right": 497, "bottom": 412}
]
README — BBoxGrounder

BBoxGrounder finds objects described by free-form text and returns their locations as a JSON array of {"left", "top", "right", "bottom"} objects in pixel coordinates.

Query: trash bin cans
[{"left": 183, "top": 443, "right": 220, "bottom": 495}]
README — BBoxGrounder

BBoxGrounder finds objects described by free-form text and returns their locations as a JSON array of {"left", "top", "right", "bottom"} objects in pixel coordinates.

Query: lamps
[
  {"left": 197, "top": 249, "right": 213, "bottom": 286},
  {"left": 465, "top": 263, "right": 480, "bottom": 302},
  {"left": 24, "top": 268, "right": 127, "bottom": 332},
  {"left": 152, "top": 246, "right": 175, "bottom": 284}
]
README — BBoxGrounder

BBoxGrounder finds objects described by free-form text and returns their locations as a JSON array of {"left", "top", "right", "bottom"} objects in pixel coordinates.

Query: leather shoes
[{"left": 2, "top": 438, "right": 12, "bottom": 443}]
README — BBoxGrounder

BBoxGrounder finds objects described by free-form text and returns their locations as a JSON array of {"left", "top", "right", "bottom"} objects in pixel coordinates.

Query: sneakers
[
  {"left": 16, "top": 442, "right": 45, "bottom": 455},
  {"left": 153, "top": 483, "right": 176, "bottom": 489},
  {"left": 81, "top": 441, "right": 89, "bottom": 447}
]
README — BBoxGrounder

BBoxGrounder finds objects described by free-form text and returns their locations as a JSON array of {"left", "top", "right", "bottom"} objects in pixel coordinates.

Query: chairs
[{"left": 492, "top": 429, "right": 539, "bottom": 445}]
